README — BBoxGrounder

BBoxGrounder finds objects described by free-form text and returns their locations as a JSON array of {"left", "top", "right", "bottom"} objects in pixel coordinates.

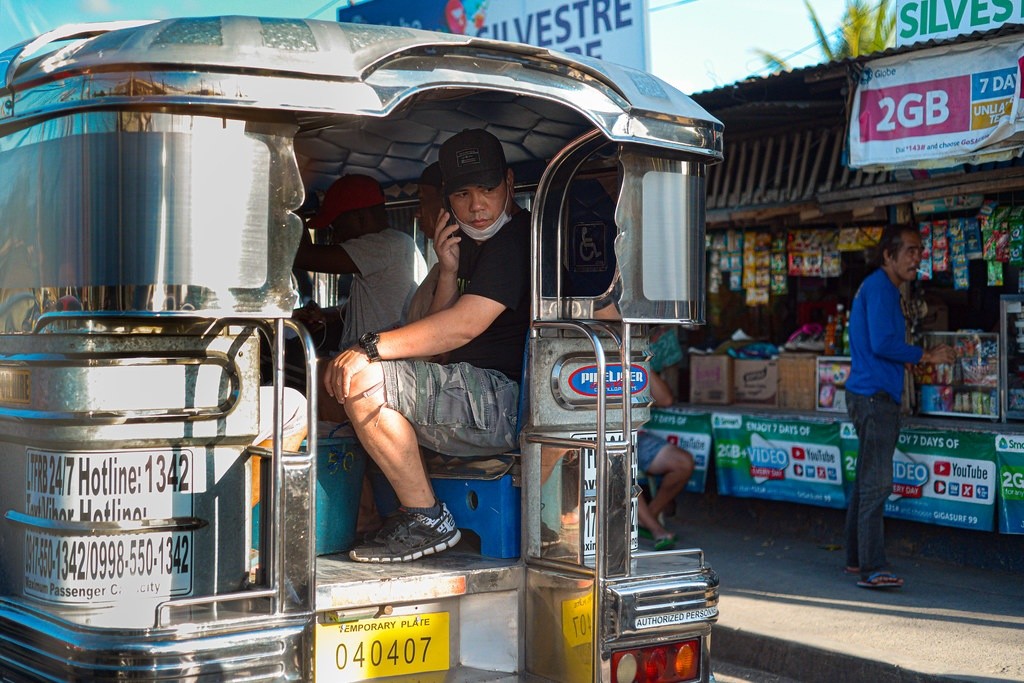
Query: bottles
[{"left": 825, "top": 305, "right": 851, "bottom": 356}]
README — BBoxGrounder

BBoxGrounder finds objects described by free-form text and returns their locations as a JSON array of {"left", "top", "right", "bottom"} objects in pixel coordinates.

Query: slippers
[
  {"left": 655, "top": 534, "right": 681, "bottom": 549},
  {"left": 844, "top": 567, "right": 859, "bottom": 574},
  {"left": 637, "top": 528, "right": 656, "bottom": 540},
  {"left": 856, "top": 572, "right": 906, "bottom": 589}
]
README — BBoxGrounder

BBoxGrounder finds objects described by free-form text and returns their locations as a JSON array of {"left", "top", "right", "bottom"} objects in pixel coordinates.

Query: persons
[
  {"left": 254, "top": 175, "right": 429, "bottom": 423},
  {"left": 637, "top": 368, "right": 696, "bottom": 552},
  {"left": 325, "top": 127, "right": 537, "bottom": 564},
  {"left": 842, "top": 225, "right": 955, "bottom": 589}
]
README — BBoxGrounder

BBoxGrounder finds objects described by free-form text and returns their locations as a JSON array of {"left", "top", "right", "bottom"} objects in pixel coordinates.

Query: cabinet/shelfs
[{"left": 918, "top": 331, "right": 1000, "bottom": 418}]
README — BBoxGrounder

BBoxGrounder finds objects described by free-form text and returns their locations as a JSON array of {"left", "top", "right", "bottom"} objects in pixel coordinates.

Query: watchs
[{"left": 359, "top": 331, "right": 382, "bottom": 362}]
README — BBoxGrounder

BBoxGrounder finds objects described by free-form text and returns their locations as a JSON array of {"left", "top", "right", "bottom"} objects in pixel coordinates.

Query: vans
[{"left": 0, "top": 15, "right": 722, "bottom": 683}]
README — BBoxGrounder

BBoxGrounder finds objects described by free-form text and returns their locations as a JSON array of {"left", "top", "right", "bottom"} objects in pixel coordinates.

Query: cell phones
[{"left": 442, "top": 194, "right": 456, "bottom": 239}]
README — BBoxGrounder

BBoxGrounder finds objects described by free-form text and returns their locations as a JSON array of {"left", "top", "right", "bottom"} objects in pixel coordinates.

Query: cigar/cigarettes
[
  {"left": 913, "top": 268, "right": 929, "bottom": 276},
  {"left": 314, "top": 320, "right": 323, "bottom": 324}
]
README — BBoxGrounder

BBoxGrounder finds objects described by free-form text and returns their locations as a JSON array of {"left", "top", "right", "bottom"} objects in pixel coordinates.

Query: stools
[{"left": 369, "top": 463, "right": 519, "bottom": 560}]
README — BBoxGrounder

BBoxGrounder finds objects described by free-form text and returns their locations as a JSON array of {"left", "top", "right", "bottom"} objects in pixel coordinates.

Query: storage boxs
[
  {"left": 689, "top": 355, "right": 735, "bottom": 405},
  {"left": 251, "top": 434, "right": 367, "bottom": 555},
  {"left": 733, "top": 360, "right": 779, "bottom": 409}
]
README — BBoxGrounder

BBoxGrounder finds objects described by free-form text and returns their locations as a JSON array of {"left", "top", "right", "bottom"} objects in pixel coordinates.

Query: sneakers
[{"left": 348, "top": 504, "right": 463, "bottom": 564}]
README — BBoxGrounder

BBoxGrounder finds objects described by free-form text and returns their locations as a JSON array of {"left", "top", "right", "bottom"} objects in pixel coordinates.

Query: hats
[
  {"left": 438, "top": 128, "right": 510, "bottom": 197},
  {"left": 306, "top": 173, "right": 389, "bottom": 231}
]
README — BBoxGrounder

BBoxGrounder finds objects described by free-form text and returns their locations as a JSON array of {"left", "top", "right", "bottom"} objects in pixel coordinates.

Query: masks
[{"left": 448, "top": 180, "right": 513, "bottom": 241}]
